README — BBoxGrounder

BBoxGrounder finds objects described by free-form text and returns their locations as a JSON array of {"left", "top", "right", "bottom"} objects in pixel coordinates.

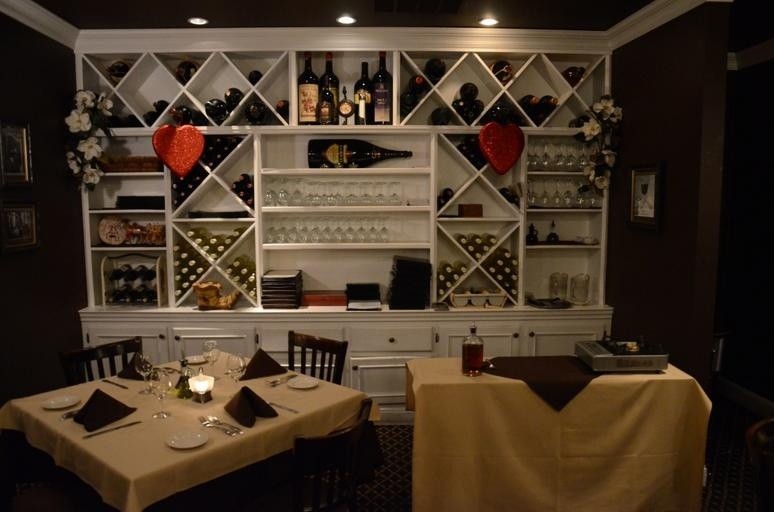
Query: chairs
[
  {"left": 287, "top": 329, "right": 347, "bottom": 387},
  {"left": 82, "top": 337, "right": 143, "bottom": 380},
  {"left": 292, "top": 419, "right": 370, "bottom": 512}
]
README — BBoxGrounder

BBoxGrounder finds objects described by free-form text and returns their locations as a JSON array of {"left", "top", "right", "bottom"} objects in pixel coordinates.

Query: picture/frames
[
  {"left": 0, "top": 122, "right": 35, "bottom": 186},
  {"left": 630, "top": 169, "right": 658, "bottom": 225},
  {"left": 0, "top": 200, "right": 41, "bottom": 253}
]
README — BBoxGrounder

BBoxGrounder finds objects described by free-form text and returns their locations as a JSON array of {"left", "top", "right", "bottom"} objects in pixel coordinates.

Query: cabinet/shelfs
[{"left": 74, "top": 28, "right": 615, "bottom": 426}]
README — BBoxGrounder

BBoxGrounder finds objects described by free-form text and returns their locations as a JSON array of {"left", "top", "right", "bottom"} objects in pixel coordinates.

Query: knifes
[
  {"left": 80, "top": 420, "right": 143, "bottom": 440},
  {"left": 102, "top": 379, "right": 128, "bottom": 391}
]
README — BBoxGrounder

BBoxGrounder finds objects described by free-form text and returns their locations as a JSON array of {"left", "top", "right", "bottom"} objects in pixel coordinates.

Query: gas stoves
[{"left": 576, "top": 330, "right": 669, "bottom": 374}]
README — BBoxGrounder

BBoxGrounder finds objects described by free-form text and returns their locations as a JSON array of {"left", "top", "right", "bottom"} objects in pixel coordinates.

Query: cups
[
  {"left": 584, "top": 233, "right": 599, "bottom": 247},
  {"left": 573, "top": 234, "right": 583, "bottom": 245},
  {"left": 571, "top": 272, "right": 589, "bottom": 306},
  {"left": 549, "top": 269, "right": 567, "bottom": 302}
]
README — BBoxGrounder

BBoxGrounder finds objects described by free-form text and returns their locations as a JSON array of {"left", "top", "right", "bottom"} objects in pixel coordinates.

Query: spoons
[{"left": 203, "top": 413, "right": 244, "bottom": 436}]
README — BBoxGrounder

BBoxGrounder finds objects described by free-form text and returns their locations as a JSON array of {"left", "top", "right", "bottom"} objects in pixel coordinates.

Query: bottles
[
  {"left": 168, "top": 135, "right": 255, "bottom": 308},
  {"left": 306, "top": 136, "right": 413, "bottom": 169},
  {"left": 402, "top": 53, "right": 595, "bottom": 126},
  {"left": 5, "top": 210, "right": 33, "bottom": 241},
  {"left": 432, "top": 130, "right": 521, "bottom": 305},
  {"left": 102, "top": 262, "right": 161, "bottom": 308},
  {"left": 98, "top": 58, "right": 288, "bottom": 126},
  {"left": 462, "top": 323, "right": 484, "bottom": 377},
  {"left": 291, "top": 48, "right": 394, "bottom": 126}
]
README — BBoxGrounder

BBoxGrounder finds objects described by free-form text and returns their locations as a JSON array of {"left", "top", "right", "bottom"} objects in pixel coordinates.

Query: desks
[{"left": 403, "top": 355, "right": 712, "bottom": 511}]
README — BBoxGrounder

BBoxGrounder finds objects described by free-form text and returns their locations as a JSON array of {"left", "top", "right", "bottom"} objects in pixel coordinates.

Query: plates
[
  {"left": 287, "top": 375, "right": 319, "bottom": 390},
  {"left": 164, "top": 425, "right": 212, "bottom": 451},
  {"left": 39, "top": 396, "right": 82, "bottom": 411}
]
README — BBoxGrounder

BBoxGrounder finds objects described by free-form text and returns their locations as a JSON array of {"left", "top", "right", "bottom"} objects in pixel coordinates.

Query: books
[
  {"left": 261, "top": 269, "right": 303, "bottom": 309},
  {"left": 346, "top": 283, "right": 382, "bottom": 311}
]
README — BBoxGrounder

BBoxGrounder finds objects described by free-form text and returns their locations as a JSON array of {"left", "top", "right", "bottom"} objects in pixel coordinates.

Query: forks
[{"left": 197, "top": 415, "right": 236, "bottom": 439}]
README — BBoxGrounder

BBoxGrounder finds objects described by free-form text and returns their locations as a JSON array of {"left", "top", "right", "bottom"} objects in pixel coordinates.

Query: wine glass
[
  {"left": 136, "top": 338, "right": 297, "bottom": 420},
  {"left": 526, "top": 138, "right": 607, "bottom": 207},
  {"left": 261, "top": 173, "right": 429, "bottom": 243}
]
